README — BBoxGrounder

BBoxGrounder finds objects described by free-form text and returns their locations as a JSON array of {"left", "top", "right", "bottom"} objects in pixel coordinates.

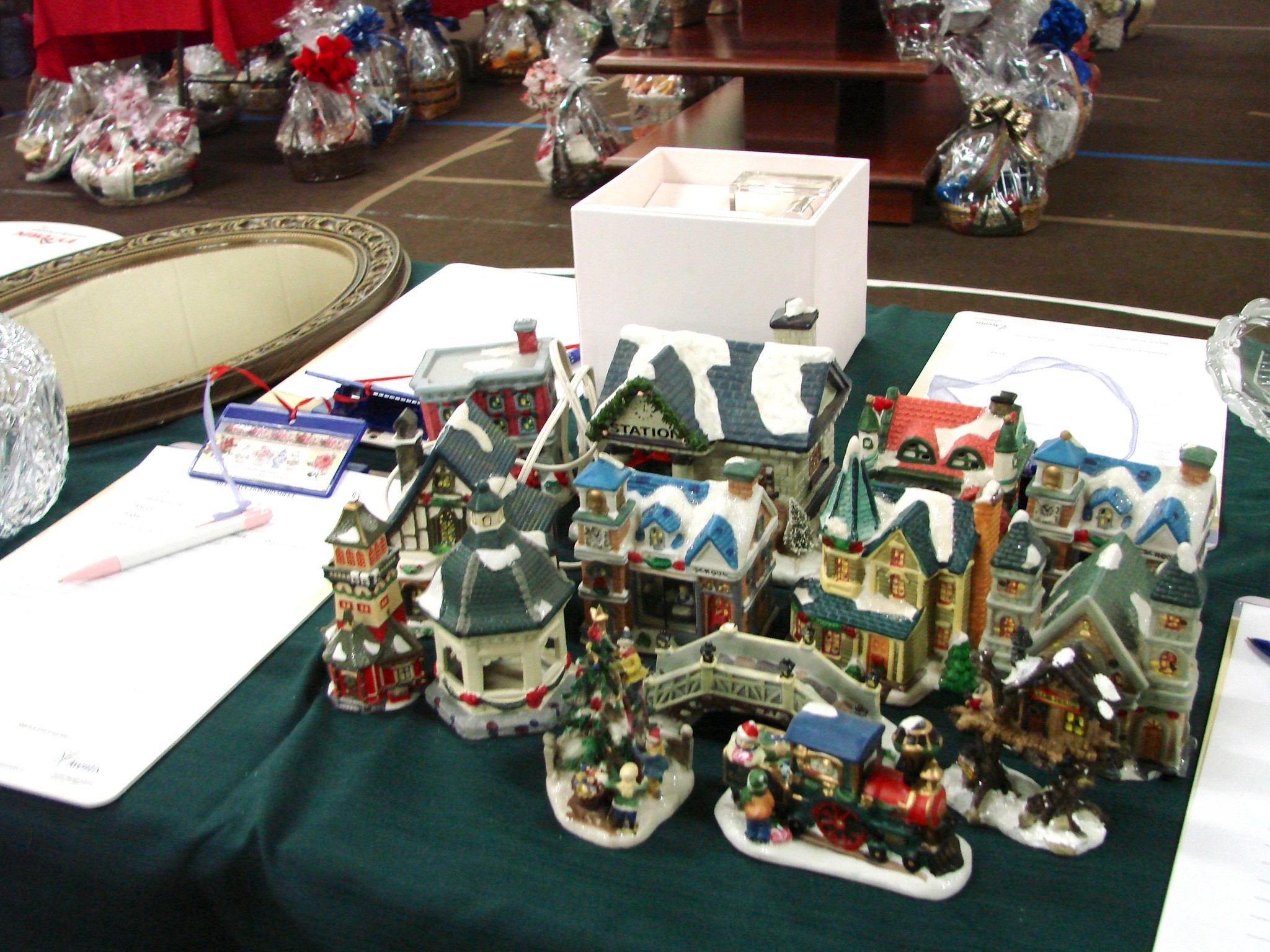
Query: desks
[
  {"left": 0, "top": 258, "right": 1270, "bottom": 952},
  {"left": 32, "top": 0, "right": 343, "bottom": 106}
]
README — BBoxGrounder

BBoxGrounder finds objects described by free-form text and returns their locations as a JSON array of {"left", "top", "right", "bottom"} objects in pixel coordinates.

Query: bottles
[{"left": 0, "top": 313, "right": 70, "bottom": 541}]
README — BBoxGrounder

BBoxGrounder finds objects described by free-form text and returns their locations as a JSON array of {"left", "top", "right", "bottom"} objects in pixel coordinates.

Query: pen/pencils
[{"left": 58, "top": 512, "right": 275, "bottom": 586}]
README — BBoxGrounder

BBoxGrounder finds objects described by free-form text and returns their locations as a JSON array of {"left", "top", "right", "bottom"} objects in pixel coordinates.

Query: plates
[{"left": 1206, "top": 297, "right": 1270, "bottom": 443}]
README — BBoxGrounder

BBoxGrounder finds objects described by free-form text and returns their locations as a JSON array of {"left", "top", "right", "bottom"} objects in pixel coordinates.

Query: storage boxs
[{"left": 569, "top": 140, "right": 873, "bottom": 376}]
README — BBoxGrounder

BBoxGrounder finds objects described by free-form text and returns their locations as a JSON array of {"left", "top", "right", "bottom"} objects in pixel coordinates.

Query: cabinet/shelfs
[{"left": 587, "top": 0, "right": 969, "bottom": 230}]
